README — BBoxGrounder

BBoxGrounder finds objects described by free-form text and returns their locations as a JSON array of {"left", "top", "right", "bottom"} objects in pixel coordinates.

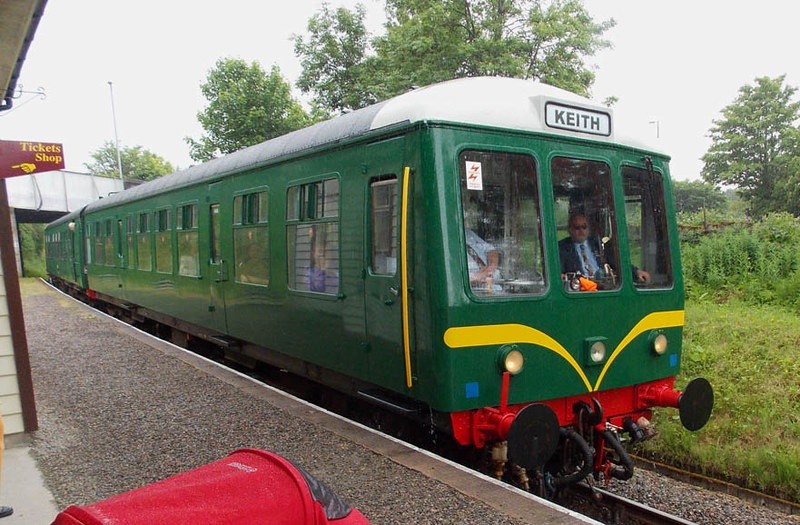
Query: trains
[{"left": 46, "top": 73, "right": 715, "bottom": 506}]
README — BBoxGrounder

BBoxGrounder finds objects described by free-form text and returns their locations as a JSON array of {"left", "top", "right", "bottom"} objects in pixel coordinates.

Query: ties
[{"left": 580, "top": 245, "right": 595, "bottom": 277}]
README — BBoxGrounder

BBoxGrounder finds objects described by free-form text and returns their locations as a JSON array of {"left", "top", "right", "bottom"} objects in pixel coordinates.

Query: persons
[
  {"left": 556, "top": 212, "right": 653, "bottom": 291},
  {"left": 463, "top": 226, "right": 504, "bottom": 295}
]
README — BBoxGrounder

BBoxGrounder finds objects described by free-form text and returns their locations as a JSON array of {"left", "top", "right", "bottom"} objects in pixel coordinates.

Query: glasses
[{"left": 570, "top": 223, "right": 589, "bottom": 230}]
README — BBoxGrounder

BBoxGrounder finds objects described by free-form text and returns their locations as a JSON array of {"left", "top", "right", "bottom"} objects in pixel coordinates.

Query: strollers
[{"left": 48, "top": 447, "right": 374, "bottom": 524}]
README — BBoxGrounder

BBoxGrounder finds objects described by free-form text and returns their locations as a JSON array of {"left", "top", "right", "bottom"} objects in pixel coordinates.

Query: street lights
[{"left": 107, "top": 80, "right": 126, "bottom": 192}]
